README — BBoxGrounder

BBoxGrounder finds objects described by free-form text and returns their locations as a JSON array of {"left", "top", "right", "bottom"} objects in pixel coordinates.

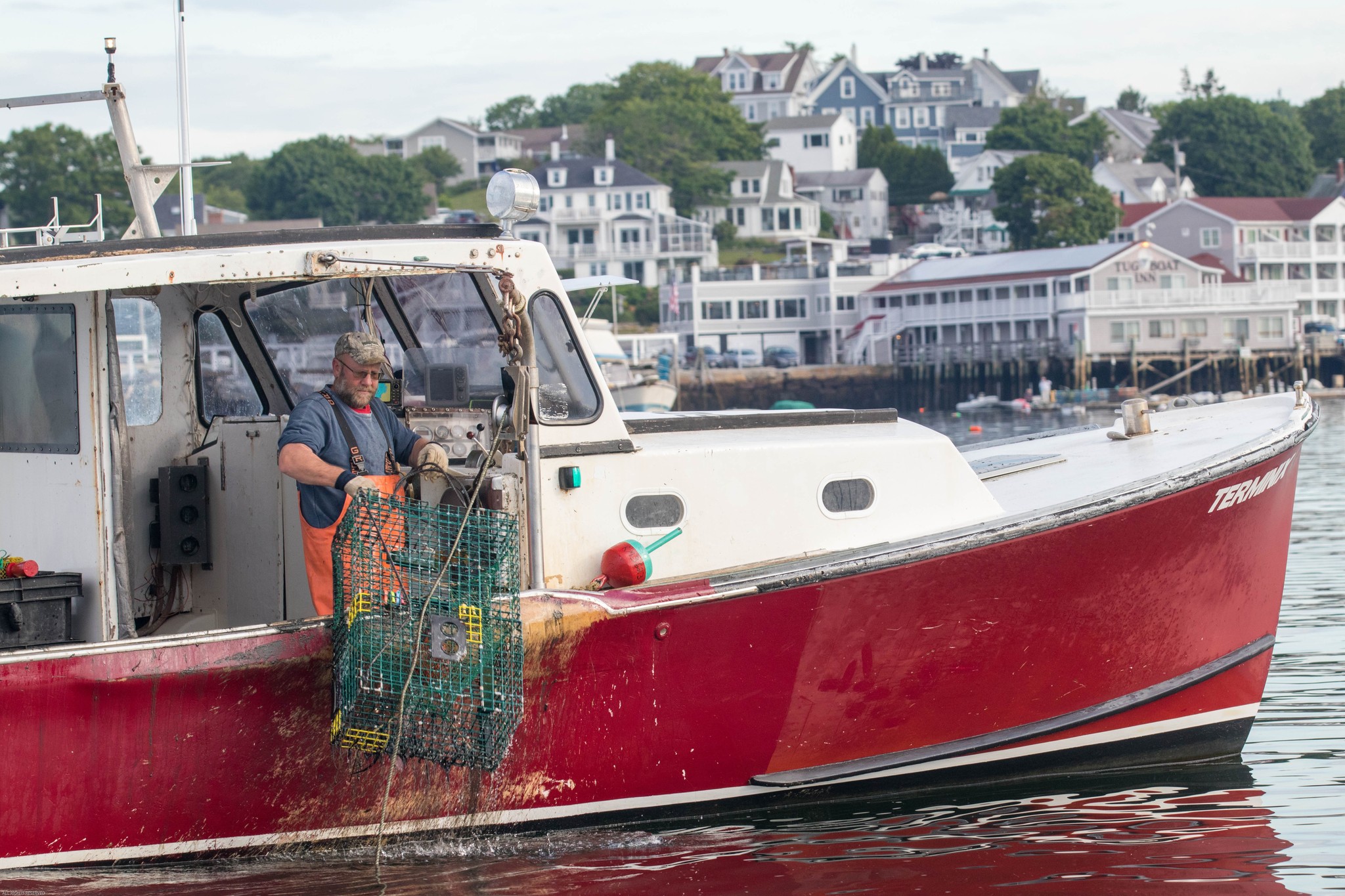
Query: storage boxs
[{"left": 0, "top": 571, "right": 82, "bottom": 651}]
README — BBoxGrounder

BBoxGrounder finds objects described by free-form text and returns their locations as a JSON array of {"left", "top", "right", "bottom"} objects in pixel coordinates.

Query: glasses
[{"left": 336, "top": 357, "right": 385, "bottom": 380}]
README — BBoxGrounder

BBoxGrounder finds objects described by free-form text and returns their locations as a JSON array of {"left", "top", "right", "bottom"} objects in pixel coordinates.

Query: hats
[{"left": 334, "top": 331, "right": 392, "bottom": 368}]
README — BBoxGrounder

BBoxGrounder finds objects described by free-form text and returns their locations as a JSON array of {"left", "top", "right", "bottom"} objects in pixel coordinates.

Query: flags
[{"left": 669, "top": 282, "right": 679, "bottom": 315}]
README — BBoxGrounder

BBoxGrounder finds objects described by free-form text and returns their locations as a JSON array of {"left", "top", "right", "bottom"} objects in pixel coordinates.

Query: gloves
[
  {"left": 335, "top": 470, "right": 379, "bottom": 504},
  {"left": 417, "top": 442, "right": 449, "bottom": 482}
]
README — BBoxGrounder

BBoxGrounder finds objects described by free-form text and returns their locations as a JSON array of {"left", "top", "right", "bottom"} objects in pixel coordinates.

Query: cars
[
  {"left": 762, "top": 344, "right": 798, "bottom": 368},
  {"left": 684, "top": 345, "right": 723, "bottom": 369},
  {"left": 721, "top": 348, "right": 760, "bottom": 368}
]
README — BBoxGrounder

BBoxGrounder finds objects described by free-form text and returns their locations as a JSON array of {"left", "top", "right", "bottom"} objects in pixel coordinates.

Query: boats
[
  {"left": 955, "top": 391, "right": 1000, "bottom": 412},
  {"left": 1, "top": 0, "right": 1323, "bottom": 879},
  {"left": 578, "top": 316, "right": 680, "bottom": 411}
]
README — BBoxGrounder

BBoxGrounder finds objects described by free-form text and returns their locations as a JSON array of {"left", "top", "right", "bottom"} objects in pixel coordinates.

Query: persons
[
  {"left": 276, "top": 331, "right": 449, "bottom": 618},
  {"left": 651, "top": 348, "right": 673, "bottom": 382},
  {"left": 1024, "top": 388, "right": 1033, "bottom": 403},
  {"left": 1038, "top": 377, "right": 1052, "bottom": 401}
]
